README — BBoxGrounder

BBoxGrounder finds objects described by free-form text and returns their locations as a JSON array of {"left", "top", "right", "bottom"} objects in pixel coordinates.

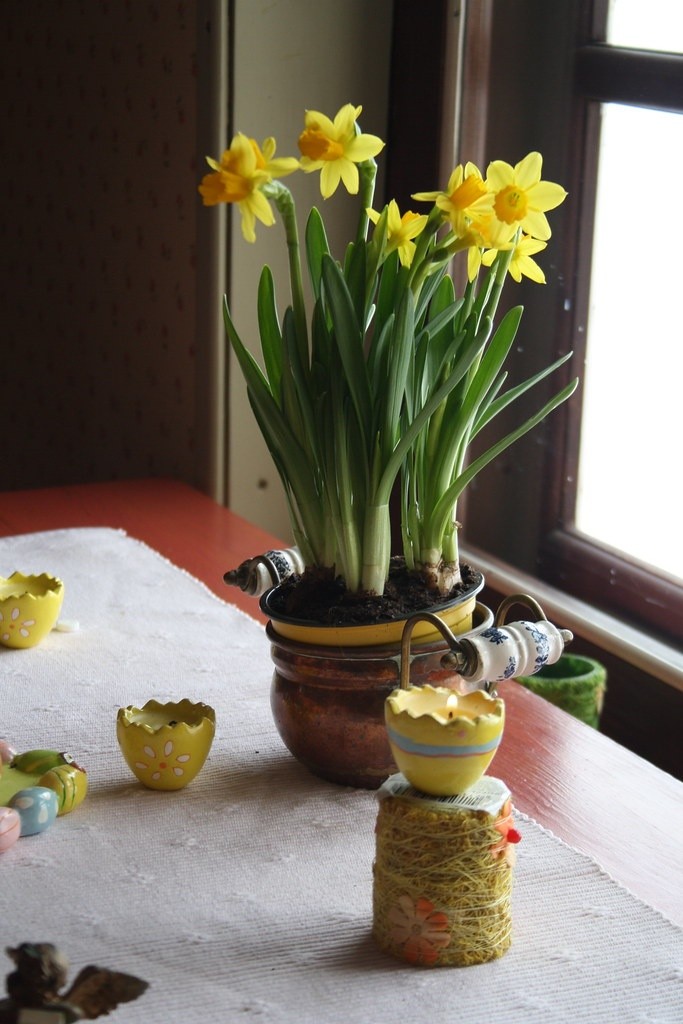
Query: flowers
[{"left": 197, "top": 101, "right": 582, "bottom": 596}]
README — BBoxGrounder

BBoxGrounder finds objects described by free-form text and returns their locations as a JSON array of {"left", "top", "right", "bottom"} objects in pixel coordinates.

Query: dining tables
[{"left": 0, "top": 479, "right": 683, "bottom": 1024}]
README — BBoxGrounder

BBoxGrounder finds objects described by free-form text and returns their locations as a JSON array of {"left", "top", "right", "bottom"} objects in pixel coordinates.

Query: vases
[{"left": 258, "top": 561, "right": 495, "bottom": 790}]
